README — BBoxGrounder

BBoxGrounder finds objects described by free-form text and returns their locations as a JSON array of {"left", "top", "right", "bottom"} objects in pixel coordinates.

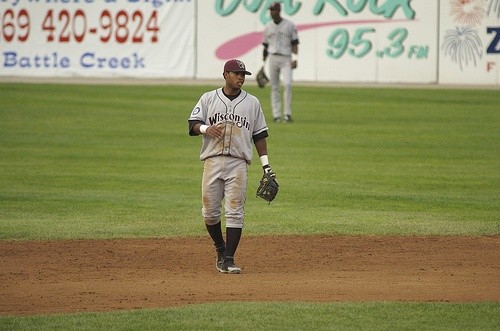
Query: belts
[{"left": 273, "top": 53, "right": 282, "bottom": 56}]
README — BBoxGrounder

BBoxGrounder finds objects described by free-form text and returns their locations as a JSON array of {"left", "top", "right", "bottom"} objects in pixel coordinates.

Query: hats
[
  {"left": 269, "top": 3, "right": 280, "bottom": 9},
  {"left": 224, "top": 59, "right": 251, "bottom": 75}
]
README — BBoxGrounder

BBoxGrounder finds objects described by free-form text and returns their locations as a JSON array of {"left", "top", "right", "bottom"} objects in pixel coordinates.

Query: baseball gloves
[
  {"left": 256, "top": 69, "right": 269, "bottom": 88},
  {"left": 255, "top": 171, "right": 280, "bottom": 204}
]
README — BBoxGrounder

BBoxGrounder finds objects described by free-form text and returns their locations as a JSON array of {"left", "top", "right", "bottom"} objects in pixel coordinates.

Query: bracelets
[
  {"left": 292, "top": 53, "right": 298, "bottom": 61},
  {"left": 260, "top": 155, "right": 269, "bottom": 166},
  {"left": 200, "top": 125, "right": 209, "bottom": 136}
]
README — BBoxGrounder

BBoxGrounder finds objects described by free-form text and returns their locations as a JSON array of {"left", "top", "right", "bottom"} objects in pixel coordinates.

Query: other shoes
[
  {"left": 274, "top": 117, "right": 282, "bottom": 123},
  {"left": 283, "top": 115, "right": 291, "bottom": 121}
]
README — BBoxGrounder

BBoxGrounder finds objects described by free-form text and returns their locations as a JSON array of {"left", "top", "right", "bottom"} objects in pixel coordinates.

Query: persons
[
  {"left": 263, "top": 2, "right": 299, "bottom": 124},
  {"left": 188, "top": 60, "right": 276, "bottom": 274}
]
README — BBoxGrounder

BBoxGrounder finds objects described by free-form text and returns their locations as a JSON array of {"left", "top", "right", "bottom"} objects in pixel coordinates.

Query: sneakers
[
  {"left": 220, "top": 259, "right": 242, "bottom": 274},
  {"left": 215, "top": 242, "right": 227, "bottom": 271}
]
